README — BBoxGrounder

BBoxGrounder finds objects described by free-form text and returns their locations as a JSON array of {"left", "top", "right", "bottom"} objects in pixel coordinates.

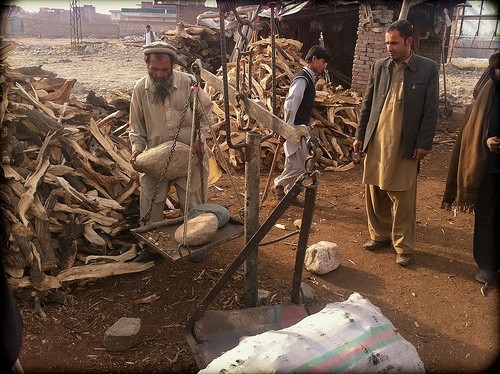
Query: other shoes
[
  {"left": 396, "top": 253, "right": 411, "bottom": 265},
  {"left": 363, "top": 239, "right": 391, "bottom": 251},
  {"left": 475, "top": 269, "right": 494, "bottom": 284},
  {"left": 133, "top": 249, "right": 162, "bottom": 262},
  {"left": 191, "top": 254, "right": 201, "bottom": 262}
]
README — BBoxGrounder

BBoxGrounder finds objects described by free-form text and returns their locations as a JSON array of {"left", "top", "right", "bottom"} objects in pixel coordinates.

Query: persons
[
  {"left": 270, "top": 45, "right": 330, "bottom": 207},
  {"left": 441, "top": 53, "right": 500, "bottom": 284},
  {"left": 353, "top": 20, "right": 439, "bottom": 266},
  {"left": 128, "top": 41, "right": 213, "bottom": 265},
  {"left": 143, "top": 25, "right": 156, "bottom": 46}
]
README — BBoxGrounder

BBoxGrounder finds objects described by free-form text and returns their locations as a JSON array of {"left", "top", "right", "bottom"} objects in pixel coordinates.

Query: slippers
[
  {"left": 271, "top": 185, "right": 285, "bottom": 200},
  {"left": 290, "top": 198, "right": 305, "bottom": 207}
]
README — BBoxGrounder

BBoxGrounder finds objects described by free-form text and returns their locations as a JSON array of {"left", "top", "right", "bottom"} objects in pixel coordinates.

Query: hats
[{"left": 143, "top": 41, "right": 177, "bottom": 60}]
